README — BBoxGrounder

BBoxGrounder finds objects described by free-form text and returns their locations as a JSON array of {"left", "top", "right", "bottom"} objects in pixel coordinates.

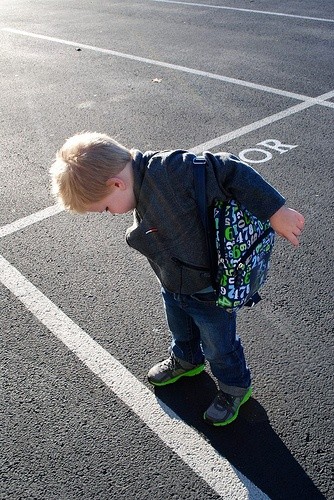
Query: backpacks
[{"left": 193, "top": 156, "right": 275, "bottom": 315}]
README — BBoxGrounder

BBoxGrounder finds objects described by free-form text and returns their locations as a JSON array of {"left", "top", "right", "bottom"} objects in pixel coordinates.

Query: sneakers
[
  {"left": 203, "top": 384, "right": 253, "bottom": 427},
  {"left": 146, "top": 357, "right": 206, "bottom": 386}
]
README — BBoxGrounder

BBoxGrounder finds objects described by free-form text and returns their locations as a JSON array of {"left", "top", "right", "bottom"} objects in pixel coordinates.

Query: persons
[{"left": 51, "top": 131, "right": 305, "bottom": 426}]
